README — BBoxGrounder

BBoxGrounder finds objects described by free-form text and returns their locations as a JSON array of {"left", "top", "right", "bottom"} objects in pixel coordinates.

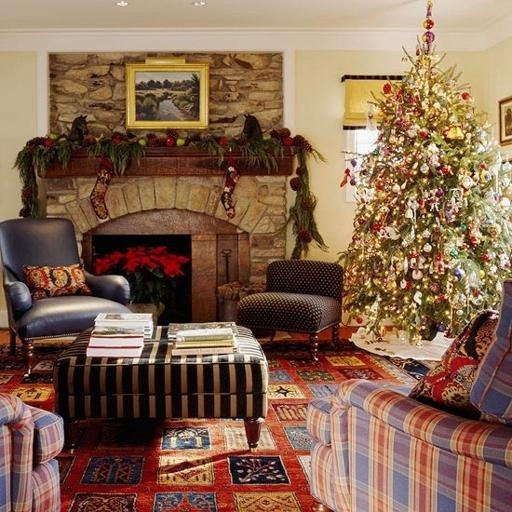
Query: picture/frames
[
  {"left": 498, "top": 96, "right": 512, "bottom": 147},
  {"left": 125, "top": 62, "right": 210, "bottom": 130}
]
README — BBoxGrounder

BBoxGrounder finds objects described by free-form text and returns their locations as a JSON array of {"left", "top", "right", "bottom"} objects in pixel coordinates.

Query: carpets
[{"left": 0, "top": 338, "right": 440, "bottom": 512}]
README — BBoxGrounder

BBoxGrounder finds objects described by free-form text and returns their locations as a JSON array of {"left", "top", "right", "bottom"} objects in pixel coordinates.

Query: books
[{"left": 85, "top": 311, "right": 240, "bottom": 359}]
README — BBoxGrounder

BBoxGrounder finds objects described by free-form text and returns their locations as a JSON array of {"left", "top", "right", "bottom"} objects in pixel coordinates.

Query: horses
[
  {"left": 243, "top": 112, "right": 264, "bottom": 142},
  {"left": 69, "top": 114, "right": 88, "bottom": 138}
]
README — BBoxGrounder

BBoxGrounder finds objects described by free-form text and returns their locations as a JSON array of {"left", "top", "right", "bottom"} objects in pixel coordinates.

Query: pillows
[
  {"left": 410, "top": 308, "right": 493, "bottom": 415},
  {"left": 22, "top": 262, "right": 91, "bottom": 299}
]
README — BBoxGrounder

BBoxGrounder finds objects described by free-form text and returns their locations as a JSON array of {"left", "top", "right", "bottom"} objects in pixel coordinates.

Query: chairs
[
  {"left": 0, "top": 217, "right": 132, "bottom": 376},
  {"left": 0, "top": 394, "right": 65, "bottom": 511},
  {"left": 307, "top": 277, "right": 512, "bottom": 512},
  {"left": 239, "top": 259, "right": 343, "bottom": 361}
]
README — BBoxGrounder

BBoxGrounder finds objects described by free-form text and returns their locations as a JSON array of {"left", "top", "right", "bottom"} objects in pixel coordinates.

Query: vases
[{"left": 127, "top": 299, "right": 165, "bottom": 325}]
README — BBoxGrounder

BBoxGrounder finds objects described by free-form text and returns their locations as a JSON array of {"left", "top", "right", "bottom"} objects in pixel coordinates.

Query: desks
[{"left": 50, "top": 318, "right": 269, "bottom": 446}]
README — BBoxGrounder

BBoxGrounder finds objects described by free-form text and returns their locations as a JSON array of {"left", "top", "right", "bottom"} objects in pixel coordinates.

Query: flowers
[{"left": 92, "top": 246, "right": 189, "bottom": 306}]
